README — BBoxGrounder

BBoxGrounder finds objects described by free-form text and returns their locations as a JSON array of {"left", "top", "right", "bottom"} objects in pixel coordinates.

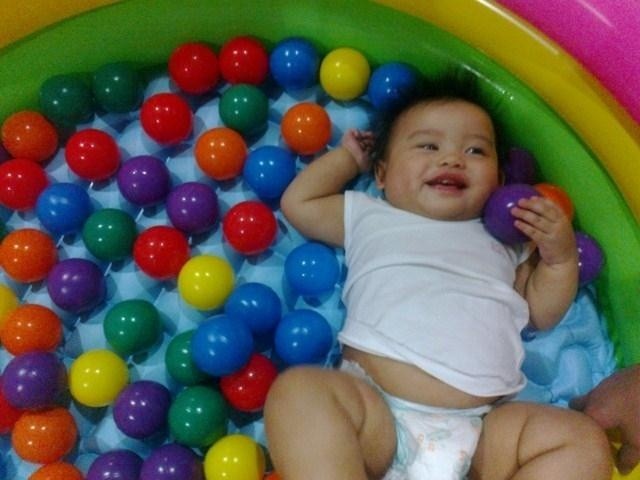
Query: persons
[
  {"left": 263, "top": 65, "right": 614, "bottom": 480},
  {"left": 569, "top": 364, "right": 639, "bottom": 475}
]
